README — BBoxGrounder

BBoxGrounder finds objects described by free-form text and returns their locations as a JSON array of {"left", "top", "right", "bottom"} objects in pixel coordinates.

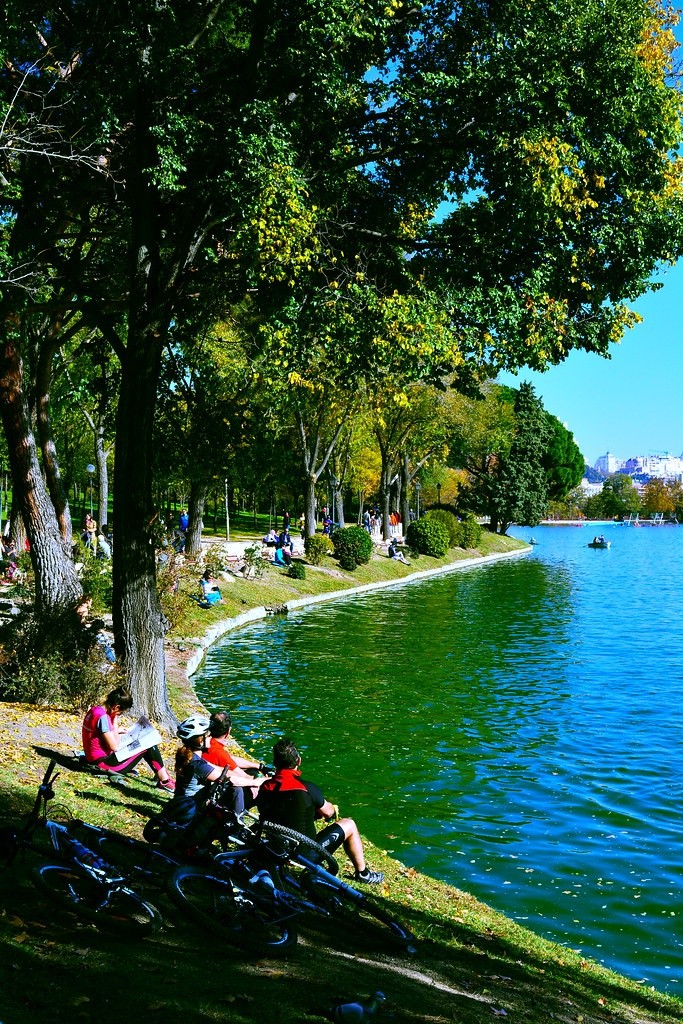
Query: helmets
[
  {"left": 161, "top": 796, "right": 196, "bottom": 824},
  {"left": 176, "top": 717, "right": 215, "bottom": 740}
]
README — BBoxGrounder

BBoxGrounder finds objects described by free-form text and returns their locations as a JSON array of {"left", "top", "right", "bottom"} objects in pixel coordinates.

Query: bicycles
[
  {"left": 6, "top": 759, "right": 169, "bottom": 936},
  {"left": 144, "top": 761, "right": 416, "bottom": 960}
]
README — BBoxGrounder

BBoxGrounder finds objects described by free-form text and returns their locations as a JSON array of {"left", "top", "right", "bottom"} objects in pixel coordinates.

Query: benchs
[{"left": 260, "top": 539, "right": 268, "bottom": 548}]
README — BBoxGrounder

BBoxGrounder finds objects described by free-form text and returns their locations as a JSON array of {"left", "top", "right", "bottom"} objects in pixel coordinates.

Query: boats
[{"left": 588, "top": 541, "right": 611, "bottom": 548}]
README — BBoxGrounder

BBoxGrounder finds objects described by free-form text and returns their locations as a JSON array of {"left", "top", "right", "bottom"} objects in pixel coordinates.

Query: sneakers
[
  {"left": 156, "top": 777, "right": 176, "bottom": 792},
  {"left": 355, "top": 870, "right": 384, "bottom": 883}
]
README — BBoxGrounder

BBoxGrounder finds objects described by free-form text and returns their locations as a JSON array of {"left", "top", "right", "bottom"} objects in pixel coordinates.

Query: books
[{"left": 114, "top": 714, "right": 161, "bottom": 762}]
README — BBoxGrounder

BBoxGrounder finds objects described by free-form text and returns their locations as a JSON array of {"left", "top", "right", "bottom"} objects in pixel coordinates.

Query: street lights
[
  {"left": 437, "top": 483, "right": 441, "bottom": 504},
  {"left": 415, "top": 481, "right": 421, "bottom": 521},
  {"left": 329, "top": 474, "right": 337, "bottom": 523},
  {"left": 87, "top": 463, "right": 95, "bottom": 518}
]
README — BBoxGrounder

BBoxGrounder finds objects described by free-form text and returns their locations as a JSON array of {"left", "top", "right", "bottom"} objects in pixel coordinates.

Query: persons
[
  {"left": 175, "top": 716, "right": 264, "bottom": 846},
  {"left": 0, "top": 535, "right": 26, "bottom": 585},
  {"left": 409, "top": 509, "right": 415, "bottom": 522},
  {"left": 257, "top": 528, "right": 294, "bottom": 567},
  {"left": 593, "top": 535, "right": 604, "bottom": 543},
  {"left": 95, "top": 534, "right": 111, "bottom": 561},
  {"left": 158, "top": 509, "right": 189, "bottom": 594},
  {"left": 202, "top": 713, "right": 271, "bottom": 810},
  {"left": 362, "top": 509, "right": 383, "bottom": 536},
  {"left": 87, "top": 619, "right": 115, "bottom": 662},
  {"left": 77, "top": 594, "right": 93, "bottom": 626},
  {"left": 199, "top": 569, "right": 226, "bottom": 605},
  {"left": 320, "top": 506, "right": 332, "bottom": 536},
  {"left": 83, "top": 687, "right": 175, "bottom": 792},
  {"left": 389, "top": 511, "right": 401, "bottom": 536},
  {"left": 259, "top": 742, "right": 384, "bottom": 883},
  {"left": 389, "top": 542, "right": 411, "bottom": 565},
  {"left": 283, "top": 512, "right": 291, "bottom": 532},
  {"left": 83, "top": 513, "right": 97, "bottom": 548}
]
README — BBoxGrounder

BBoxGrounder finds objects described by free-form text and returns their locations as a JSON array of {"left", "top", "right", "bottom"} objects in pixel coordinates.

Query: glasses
[{"left": 202, "top": 731, "right": 212, "bottom": 740}]
